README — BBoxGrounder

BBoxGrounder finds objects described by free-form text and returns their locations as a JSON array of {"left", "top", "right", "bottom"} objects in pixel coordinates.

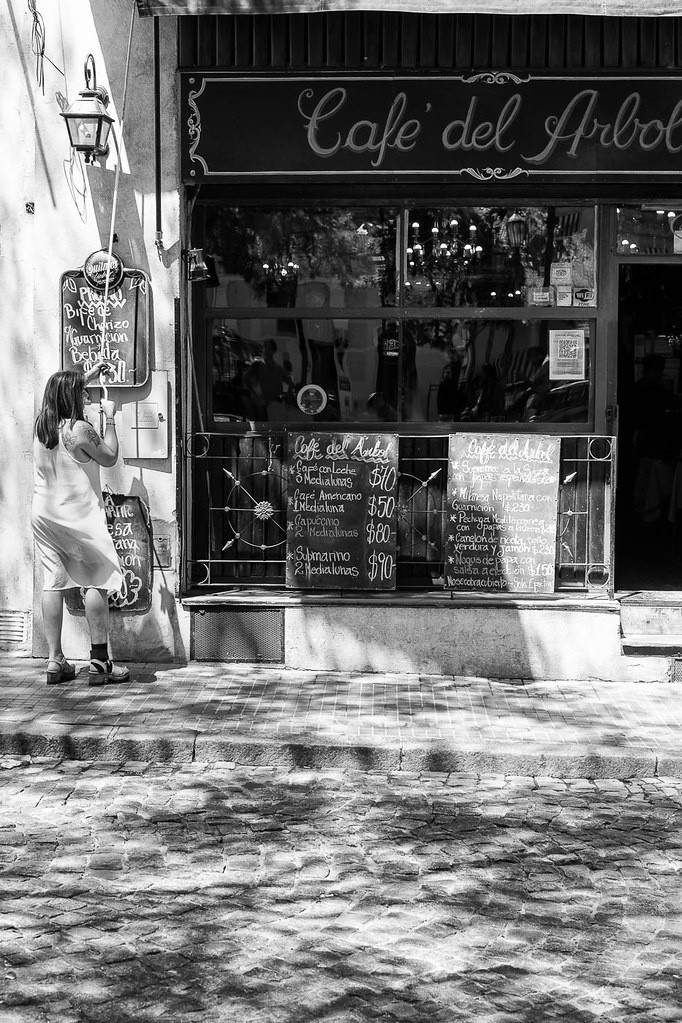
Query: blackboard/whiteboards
[
  {"left": 285, "top": 433, "right": 400, "bottom": 589},
  {"left": 57, "top": 267, "right": 150, "bottom": 388},
  {"left": 64, "top": 492, "right": 153, "bottom": 613},
  {"left": 443, "top": 432, "right": 562, "bottom": 594}
]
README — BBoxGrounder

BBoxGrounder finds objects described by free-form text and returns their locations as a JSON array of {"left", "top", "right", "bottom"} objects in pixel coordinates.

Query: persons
[
  {"left": 618, "top": 354, "right": 673, "bottom": 536},
  {"left": 376, "top": 321, "right": 418, "bottom": 422},
  {"left": 31, "top": 363, "right": 130, "bottom": 686}
]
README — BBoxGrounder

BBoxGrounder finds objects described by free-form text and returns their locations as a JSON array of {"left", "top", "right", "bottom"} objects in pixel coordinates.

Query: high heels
[
  {"left": 88, "top": 659, "right": 130, "bottom": 686},
  {"left": 45, "top": 657, "right": 75, "bottom": 685}
]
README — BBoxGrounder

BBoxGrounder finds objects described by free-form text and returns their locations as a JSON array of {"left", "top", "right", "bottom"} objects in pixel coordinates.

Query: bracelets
[
  {"left": 84, "top": 372, "right": 90, "bottom": 383},
  {"left": 107, "top": 418, "right": 113, "bottom": 419},
  {"left": 106, "top": 423, "right": 116, "bottom": 425}
]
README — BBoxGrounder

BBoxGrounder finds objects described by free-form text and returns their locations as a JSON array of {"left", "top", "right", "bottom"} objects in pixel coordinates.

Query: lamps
[{"left": 59, "top": 52, "right": 115, "bottom": 164}]
